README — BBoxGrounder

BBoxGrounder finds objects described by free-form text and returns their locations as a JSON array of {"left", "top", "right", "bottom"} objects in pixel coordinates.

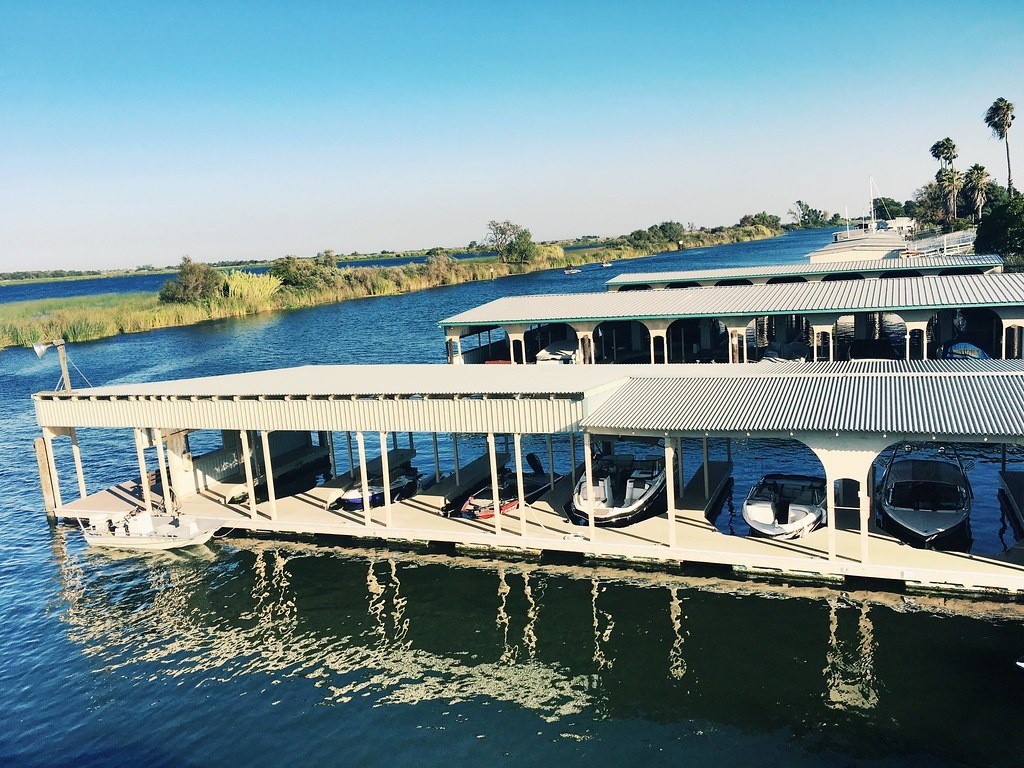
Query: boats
[
  {"left": 460, "top": 454, "right": 565, "bottom": 521},
  {"left": 759, "top": 340, "right": 811, "bottom": 363},
  {"left": 563, "top": 269, "right": 581, "bottom": 275},
  {"left": 875, "top": 441, "right": 975, "bottom": 550},
  {"left": 601, "top": 261, "right": 612, "bottom": 267},
  {"left": 741, "top": 473, "right": 827, "bottom": 541},
  {"left": 535, "top": 338, "right": 579, "bottom": 365},
  {"left": 78, "top": 508, "right": 227, "bottom": 552},
  {"left": 340, "top": 471, "right": 448, "bottom": 510},
  {"left": 845, "top": 338, "right": 901, "bottom": 362},
  {"left": 571, "top": 438, "right": 678, "bottom": 528}
]
